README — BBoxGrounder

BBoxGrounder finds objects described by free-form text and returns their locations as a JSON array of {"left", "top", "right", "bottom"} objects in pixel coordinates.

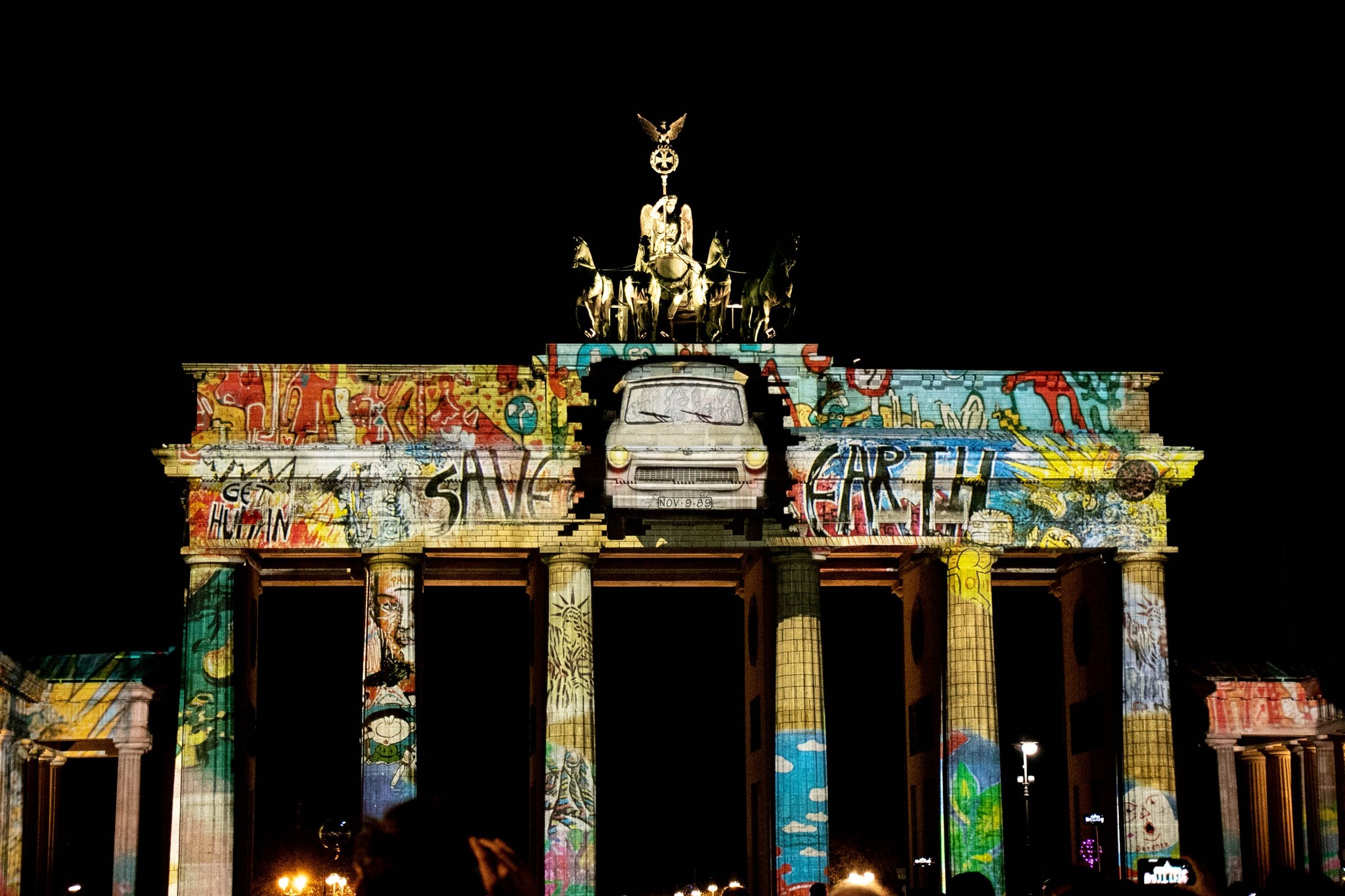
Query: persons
[
  {"left": 648, "top": 194, "right": 684, "bottom": 255},
  {"left": 355, "top": 788, "right": 489, "bottom": 896},
  {"left": 948, "top": 871, "right": 996, "bottom": 896},
  {"left": 810, "top": 883, "right": 827, "bottom": 896}
]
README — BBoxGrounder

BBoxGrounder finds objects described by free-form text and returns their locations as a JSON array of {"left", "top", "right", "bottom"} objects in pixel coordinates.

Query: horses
[
  {"left": 570, "top": 236, "right": 614, "bottom": 341},
  {"left": 624, "top": 234, "right": 662, "bottom": 343},
  {"left": 740, "top": 232, "right": 802, "bottom": 343},
  {"left": 703, "top": 230, "right": 731, "bottom": 344}
]
